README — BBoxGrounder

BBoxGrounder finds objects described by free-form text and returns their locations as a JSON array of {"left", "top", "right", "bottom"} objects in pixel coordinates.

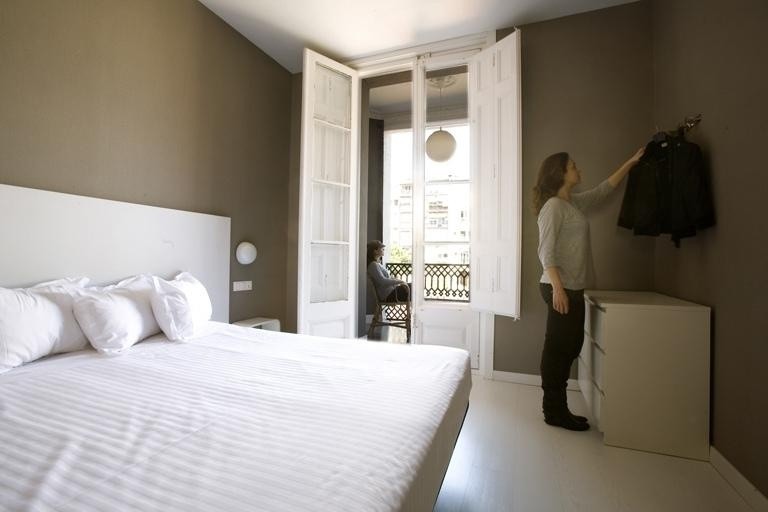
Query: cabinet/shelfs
[{"left": 578, "top": 291, "right": 711, "bottom": 461}]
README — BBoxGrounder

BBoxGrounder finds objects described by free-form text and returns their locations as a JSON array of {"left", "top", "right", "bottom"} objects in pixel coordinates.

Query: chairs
[{"left": 366, "top": 275, "right": 411, "bottom": 342}]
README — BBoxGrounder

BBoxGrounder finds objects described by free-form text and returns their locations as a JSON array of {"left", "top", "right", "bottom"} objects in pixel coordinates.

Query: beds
[{"left": 0, "top": 183, "right": 472, "bottom": 512}]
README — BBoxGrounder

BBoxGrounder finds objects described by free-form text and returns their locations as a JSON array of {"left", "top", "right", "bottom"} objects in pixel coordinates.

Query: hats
[{"left": 367, "top": 240, "right": 385, "bottom": 249}]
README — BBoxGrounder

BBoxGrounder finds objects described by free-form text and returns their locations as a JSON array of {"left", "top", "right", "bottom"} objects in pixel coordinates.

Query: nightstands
[{"left": 232, "top": 317, "right": 280, "bottom": 331}]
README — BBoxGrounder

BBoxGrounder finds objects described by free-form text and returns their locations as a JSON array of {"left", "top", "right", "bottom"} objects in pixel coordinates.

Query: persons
[
  {"left": 531, "top": 148, "right": 644, "bottom": 431},
  {"left": 366, "top": 239, "right": 412, "bottom": 302}
]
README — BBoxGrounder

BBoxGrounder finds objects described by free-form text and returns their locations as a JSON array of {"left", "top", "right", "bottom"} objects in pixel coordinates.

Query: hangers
[{"left": 642, "top": 123, "right": 691, "bottom": 161}]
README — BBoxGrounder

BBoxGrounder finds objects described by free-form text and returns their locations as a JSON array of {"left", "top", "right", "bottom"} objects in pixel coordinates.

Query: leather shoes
[{"left": 545, "top": 413, "right": 589, "bottom": 430}]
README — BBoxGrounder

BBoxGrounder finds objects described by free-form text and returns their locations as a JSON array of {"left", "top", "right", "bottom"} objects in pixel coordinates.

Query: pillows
[
  {"left": 70, "top": 271, "right": 166, "bottom": 356},
  {"left": 0, "top": 273, "right": 98, "bottom": 374},
  {"left": 151, "top": 271, "right": 212, "bottom": 342}
]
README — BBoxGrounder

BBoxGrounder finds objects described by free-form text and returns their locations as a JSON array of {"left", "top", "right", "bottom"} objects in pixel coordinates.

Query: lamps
[
  {"left": 237, "top": 242, "right": 257, "bottom": 265},
  {"left": 426, "top": 76, "right": 456, "bottom": 162}
]
